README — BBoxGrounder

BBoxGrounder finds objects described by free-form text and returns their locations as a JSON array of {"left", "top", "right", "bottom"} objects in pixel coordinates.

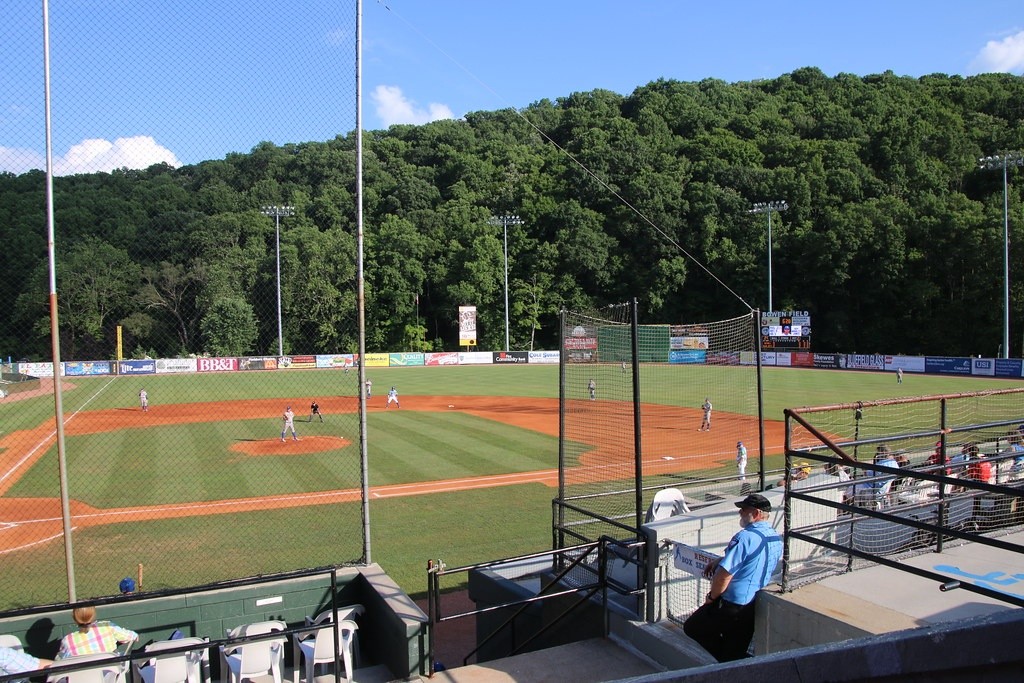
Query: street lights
[
  {"left": 746, "top": 200, "right": 789, "bottom": 313},
  {"left": 978, "top": 153, "right": 1024, "bottom": 361},
  {"left": 485, "top": 215, "right": 525, "bottom": 352},
  {"left": 260, "top": 205, "right": 295, "bottom": 357}
]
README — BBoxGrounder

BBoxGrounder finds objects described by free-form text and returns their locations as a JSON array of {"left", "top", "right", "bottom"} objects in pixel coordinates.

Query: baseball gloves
[{"left": 283, "top": 414, "right": 288, "bottom": 421}]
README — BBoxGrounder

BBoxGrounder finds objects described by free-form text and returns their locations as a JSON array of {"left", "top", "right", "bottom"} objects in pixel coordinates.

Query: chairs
[
  {"left": 647, "top": 448, "right": 1024, "bottom": 540},
  {"left": 305, "top": 604, "right": 368, "bottom": 674},
  {"left": 133, "top": 635, "right": 210, "bottom": 683},
  {"left": 293, "top": 620, "right": 359, "bottom": 683},
  {"left": 47, "top": 652, "right": 129, "bottom": 683},
  {"left": 221, "top": 638, "right": 283, "bottom": 683},
  {"left": 227, "top": 618, "right": 287, "bottom": 680}
]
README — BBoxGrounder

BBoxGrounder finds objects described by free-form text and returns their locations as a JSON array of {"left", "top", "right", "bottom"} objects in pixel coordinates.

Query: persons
[
  {"left": 622, "top": 361, "right": 626, "bottom": 372},
  {"left": 896, "top": 367, "right": 903, "bottom": 384},
  {"left": 366, "top": 378, "right": 372, "bottom": 398},
  {"left": 308, "top": 401, "right": 324, "bottom": 423},
  {"left": 119, "top": 577, "right": 136, "bottom": 594},
  {"left": 0, "top": 646, "right": 55, "bottom": 683},
  {"left": 588, "top": 379, "right": 596, "bottom": 401},
  {"left": 842, "top": 425, "right": 1024, "bottom": 515},
  {"left": 139, "top": 389, "right": 148, "bottom": 412},
  {"left": 282, "top": 406, "right": 298, "bottom": 442},
  {"left": 683, "top": 493, "right": 784, "bottom": 663},
  {"left": 55, "top": 602, "right": 139, "bottom": 683},
  {"left": 698, "top": 398, "right": 712, "bottom": 432},
  {"left": 782, "top": 326, "right": 791, "bottom": 336},
  {"left": 385, "top": 386, "right": 401, "bottom": 408},
  {"left": 736, "top": 442, "right": 747, "bottom": 479}
]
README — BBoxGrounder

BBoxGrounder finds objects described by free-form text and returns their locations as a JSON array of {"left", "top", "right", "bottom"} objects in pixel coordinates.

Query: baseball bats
[{"left": 138, "top": 563, "right": 142, "bottom": 591}]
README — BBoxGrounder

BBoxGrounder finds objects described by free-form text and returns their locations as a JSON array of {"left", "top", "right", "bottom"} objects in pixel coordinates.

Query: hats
[
  {"left": 737, "top": 442, "right": 742, "bottom": 446},
  {"left": 734, "top": 494, "right": 772, "bottom": 512},
  {"left": 287, "top": 406, "right": 291, "bottom": 410},
  {"left": 312, "top": 401, "right": 315, "bottom": 404}
]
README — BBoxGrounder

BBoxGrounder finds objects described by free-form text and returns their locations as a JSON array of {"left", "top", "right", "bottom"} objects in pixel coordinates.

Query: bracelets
[{"left": 706, "top": 591, "right": 712, "bottom": 600}]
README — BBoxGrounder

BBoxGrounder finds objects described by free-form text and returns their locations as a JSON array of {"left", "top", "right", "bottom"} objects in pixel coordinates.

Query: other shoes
[
  {"left": 705, "top": 429, "right": 709, "bottom": 431},
  {"left": 282, "top": 440, "right": 286, "bottom": 442},
  {"left": 294, "top": 438, "right": 298, "bottom": 441},
  {"left": 321, "top": 420, "right": 324, "bottom": 422},
  {"left": 309, "top": 421, "right": 311, "bottom": 423},
  {"left": 697, "top": 429, "right": 703, "bottom": 431}
]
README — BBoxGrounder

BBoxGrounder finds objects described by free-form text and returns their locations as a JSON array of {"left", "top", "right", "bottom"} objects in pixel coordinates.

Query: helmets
[{"left": 119, "top": 578, "right": 135, "bottom": 593}]
